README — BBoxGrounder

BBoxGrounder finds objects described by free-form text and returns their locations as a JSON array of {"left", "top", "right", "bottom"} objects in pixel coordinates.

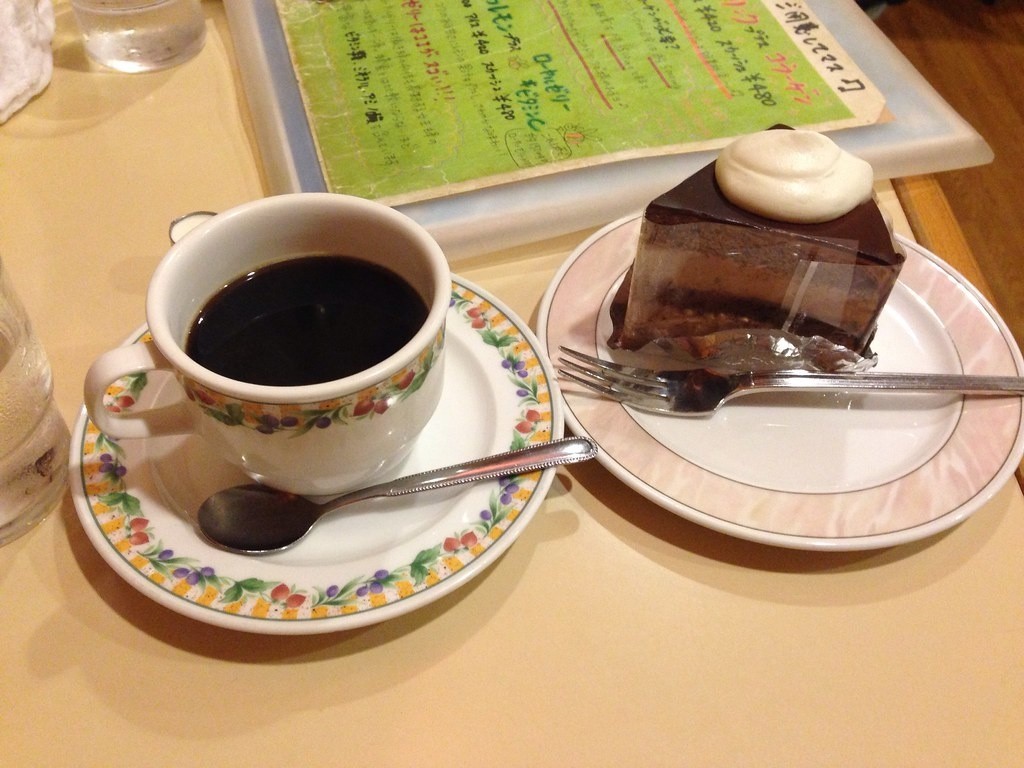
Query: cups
[
  {"left": 0, "top": 259, "right": 72, "bottom": 551},
  {"left": 84, "top": 192, "right": 452, "bottom": 495},
  {"left": 70, "top": 0, "right": 208, "bottom": 74}
]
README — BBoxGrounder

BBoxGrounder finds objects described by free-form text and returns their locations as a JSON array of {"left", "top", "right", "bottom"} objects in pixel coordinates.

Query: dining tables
[{"left": 0, "top": 0, "right": 1024, "bottom": 766}]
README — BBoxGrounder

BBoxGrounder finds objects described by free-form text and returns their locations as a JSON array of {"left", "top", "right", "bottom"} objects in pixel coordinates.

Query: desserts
[{"left": 616, "top": 122, "right": 906, "bottom": 359}]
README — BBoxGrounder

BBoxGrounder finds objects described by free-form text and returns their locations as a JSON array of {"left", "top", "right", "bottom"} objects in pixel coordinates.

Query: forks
[{"left": 557, "top": 344, "right": 1024, "bottom": 417}]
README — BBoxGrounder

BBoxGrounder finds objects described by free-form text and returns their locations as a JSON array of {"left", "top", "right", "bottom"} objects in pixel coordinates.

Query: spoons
[{"left": 196, "top": 435, "right": 597, "bottom": 554}]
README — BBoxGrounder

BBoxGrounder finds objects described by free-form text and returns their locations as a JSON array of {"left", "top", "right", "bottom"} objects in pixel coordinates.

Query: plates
[
  {"left": 536, "top": 208, "right": 1024, "bottom": 552},
  {"left": 68, "top": 271, "right": 563, "bottom": 636}
]
